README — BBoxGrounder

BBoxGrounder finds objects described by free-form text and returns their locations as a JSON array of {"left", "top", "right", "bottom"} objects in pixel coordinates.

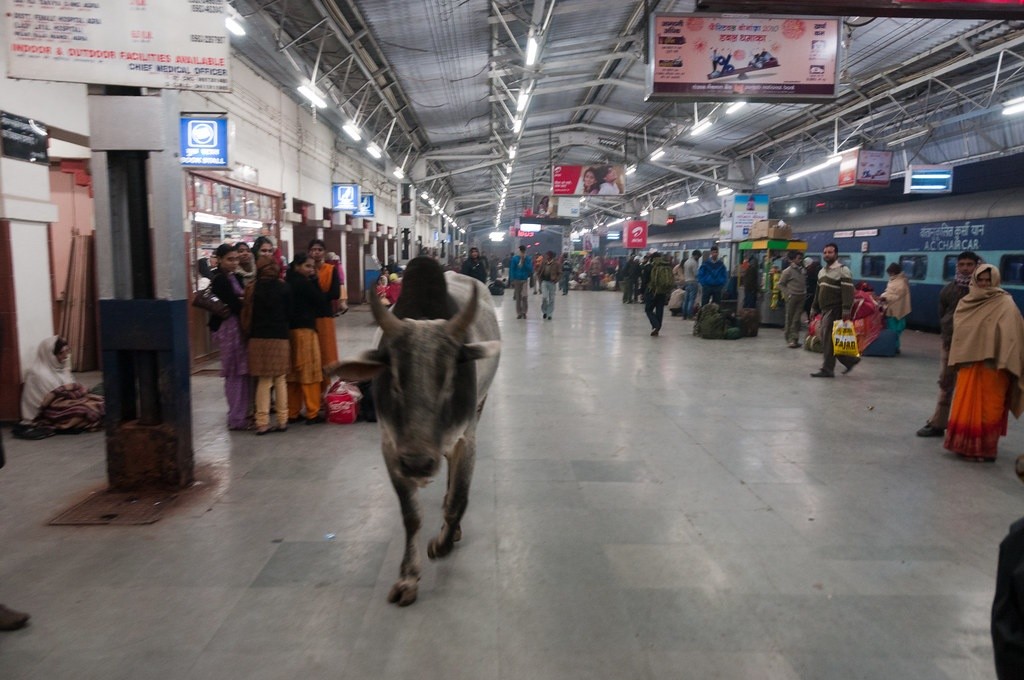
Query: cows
[{"left": 333, "top": 271, "right": 503, "bottom": 607}]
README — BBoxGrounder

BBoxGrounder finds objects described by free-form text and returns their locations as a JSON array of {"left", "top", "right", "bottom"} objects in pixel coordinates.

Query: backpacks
[
  {"left": 648, "top": 258, "right": 676, "bottom": 297},
  {"left": 562, "top": 258, "right": 571, "bottom": 272}
]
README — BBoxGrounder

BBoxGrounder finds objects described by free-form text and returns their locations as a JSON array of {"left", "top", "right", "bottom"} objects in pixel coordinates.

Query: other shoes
[
  {"left": 289, "top": 414, "right": 305, "bottom": 423},
  {"left": 544, "top": 313, "right": 548, "bottom": 319},
  {"left": 548, "top": 315, "right": 552, "bottom": 319},
  {"left": 916, "top": 423, "right": 944, "bottom": 437},
  {"left": 517, "top": 313, "right": 526, "bottom": 320},
  {"left": 651, "top": 327, "right": 659, "bottom": 336},
  {"left": 843, "top": 357, "right": 863, "bottom": 375},
  {"left": 307, "top": 415, "right": 324, "bottom": 424},
  {"left": 789, "top": 340, "right": 801, "bottom": 347},
  {"left": 810, "top": 367, "right": 834, "bottom": 378}
]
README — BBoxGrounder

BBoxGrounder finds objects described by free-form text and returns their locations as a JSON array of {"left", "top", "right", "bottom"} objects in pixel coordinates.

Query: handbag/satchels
[
  {"left": 489, "top": 282, "right": 503, "bottom": 295},
  {"left": 696, "top": 302, "right": 760, "bottom": 340},
  {"left": 832, "top": 318, "right": 861, "bottom": 359},
  {"left": 193, "top": 273, "right": 233, "bottom": 320},
  {"left": 323, "top": 380, "right": 363, "bottom": 424}
]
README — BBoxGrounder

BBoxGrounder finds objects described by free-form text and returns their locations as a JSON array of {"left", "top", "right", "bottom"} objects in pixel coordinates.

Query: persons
[
  {"left": 917, "top": 251, "right": 986, "bottom": 438},
  {"left": 194, "top": 233, "right": 349, "bottom": 436},
  {"left": 746, "top": 197, "right": 755, "bottom": 209},
  {"left": 583, "top": 167, "right": 599, "bottom": 195},
  {"left": 803, "top": 257, "right": 822, "bottom": 312},
  {"left": 878, "top": 263, "right": 912, "bottom": 353},
  {"left": 942, "top": 264, "right": 1024, "bottom": 461},
  {"left": 778, "top": 252, "right": 807, "bottom": 348},
  {"left": 601, "top": 166, "right": 620, "bottom": 194},
  {"left": 461, "top": 245, "right": 732, "bottom": 338},
  {"left": 0, "top": 432, "right": 31, "bottom": 630},
  {"left": 537, "top": 196, "right": 549, "bottom": 217},
  {"left": 376, "top": 262, "right": 404, "bottom": 305},
  {"left": 741, "top": 258, "right": 758, "bottom": 308},
  {"left": 22, "top": 335, "right": 104, "bottom": 432},
  {"left": 808, "top": 243, "right": 853, "bottom": 377}
]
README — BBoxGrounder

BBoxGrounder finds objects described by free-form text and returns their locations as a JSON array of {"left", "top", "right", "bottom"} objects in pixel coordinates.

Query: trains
[{"left": 603, "top": 182, "right": 1024, "bottom": 333}]
